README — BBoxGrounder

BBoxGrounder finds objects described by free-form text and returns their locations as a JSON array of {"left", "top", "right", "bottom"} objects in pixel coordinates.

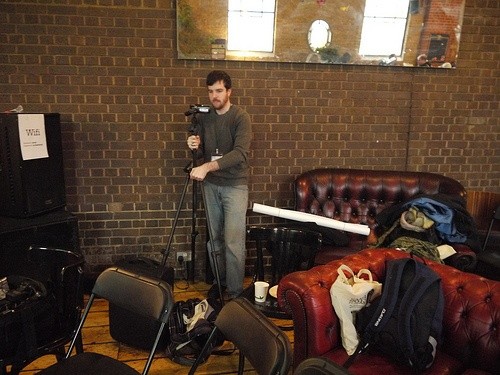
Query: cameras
[{"left": 189, "top": 103, "right": 211, "bottom": 114}]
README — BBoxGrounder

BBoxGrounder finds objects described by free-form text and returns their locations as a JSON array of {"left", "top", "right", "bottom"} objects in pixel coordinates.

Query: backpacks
[{"left": 343, "top": 257, "right": 445, "bottom": 373}]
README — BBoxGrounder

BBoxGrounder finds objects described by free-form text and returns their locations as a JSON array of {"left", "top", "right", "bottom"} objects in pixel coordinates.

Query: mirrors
[{"left": 176, "top": 0, "right": 466, "bottom": 69}]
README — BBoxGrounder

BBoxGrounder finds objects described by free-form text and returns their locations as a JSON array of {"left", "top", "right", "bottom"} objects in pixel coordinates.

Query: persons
[
  {"left": 417, "top": 54, "right": 432, "bottom": 67},
  {"left": 305, "top": 53, "right": 321, "bottom": 63},
  {"left": 186, "top": 69, "right": 254, "bottom": 307}
]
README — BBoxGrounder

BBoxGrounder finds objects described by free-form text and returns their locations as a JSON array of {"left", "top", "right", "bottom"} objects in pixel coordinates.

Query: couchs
[
  {"left": 294, "top": 168, "right": 477, "bottom": 273},
  {"left": 277, "top": 248, "right": 500, "bottom": 375}
]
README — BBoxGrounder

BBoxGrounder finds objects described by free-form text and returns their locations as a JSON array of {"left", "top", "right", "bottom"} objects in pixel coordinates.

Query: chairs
[
  {"left": 32, "top": 267, "right": 172, "bottom": 375},
  {"left": 0, "top": 246, "right": 85, "bottom": 375},
  {"left": 482, "top": 204, "right": 500, "bottom": 253},
  {"left": 239, "top": 228, "right": 323, "bottom": 375},
  {"left": 189, "top": 296, "right": 292, "bottom": 375}
]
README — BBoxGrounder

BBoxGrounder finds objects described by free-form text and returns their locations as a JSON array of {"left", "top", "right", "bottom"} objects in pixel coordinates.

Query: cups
[{"left": 254, "top": 281, "right": 270, "bottom": 303}]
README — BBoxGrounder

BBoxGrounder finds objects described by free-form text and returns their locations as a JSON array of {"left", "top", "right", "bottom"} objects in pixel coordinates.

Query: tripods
[{"left": 160, "top": 115, "right": 225, "bottom": 308}]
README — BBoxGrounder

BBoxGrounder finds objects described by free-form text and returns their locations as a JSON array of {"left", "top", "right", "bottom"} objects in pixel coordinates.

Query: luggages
[{"left": 108, "top": 255, "right": 175, "bottom": 350}]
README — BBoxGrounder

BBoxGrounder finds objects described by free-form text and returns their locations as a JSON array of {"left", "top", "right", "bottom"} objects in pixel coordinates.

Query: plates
[{"left": 269, "top": 285, "right": 278, "bottom": 298}]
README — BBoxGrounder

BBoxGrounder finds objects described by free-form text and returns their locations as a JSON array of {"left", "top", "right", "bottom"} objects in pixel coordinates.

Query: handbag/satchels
[
  {"left": 169, "top": 297, "right": 219, "bottom": 356},
  {"left": 330, "top": 263, "right": 382, "bottom": 356}
]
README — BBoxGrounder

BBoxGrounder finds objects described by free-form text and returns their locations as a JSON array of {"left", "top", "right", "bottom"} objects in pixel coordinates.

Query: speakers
[
  {"left": 0, "top": 208, "right": 83, "bottom": 366},
  {"left": 109, "top": 254, "right": 174, "bottom": 349},
  {"left": 0, "top": 112, "right": 67, "bottom": 218}
]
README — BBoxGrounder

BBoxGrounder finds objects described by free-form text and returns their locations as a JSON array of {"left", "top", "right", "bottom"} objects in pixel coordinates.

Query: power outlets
[{"left": 177, "top": 251, "right": 189, "bottom": 262}]
